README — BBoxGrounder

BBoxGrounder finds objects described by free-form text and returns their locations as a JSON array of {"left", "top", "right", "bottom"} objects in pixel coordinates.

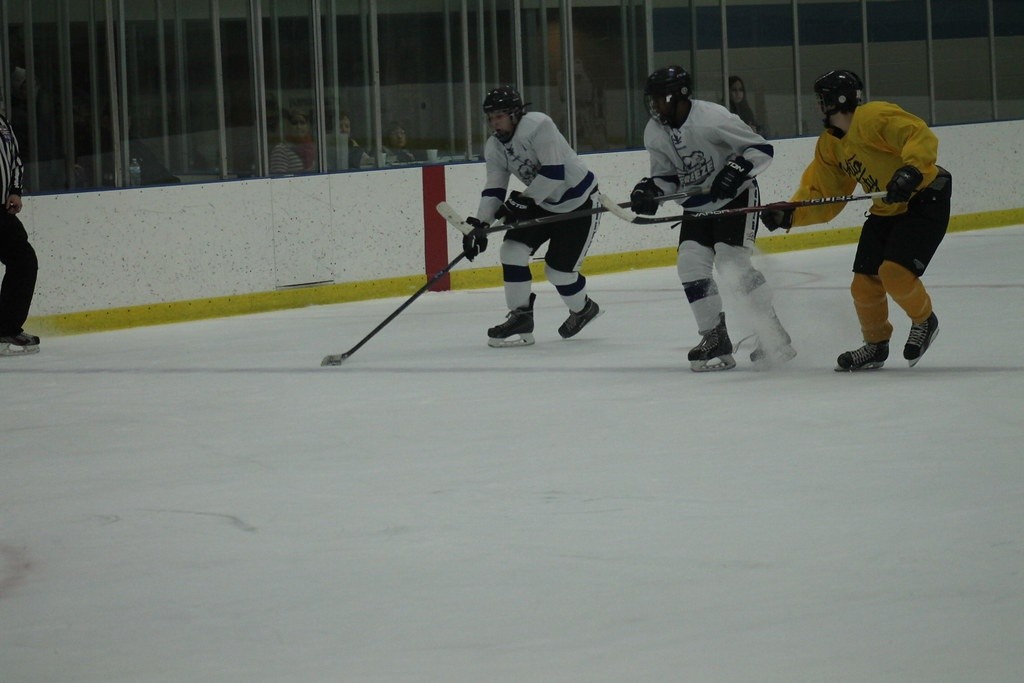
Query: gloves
[
  {"left": 760, "top": 201, "right": 794, "bottom": 232},
  {"left": 494, "top": 191, "right": 536, "bottom": 225},
  {"left": 463, "top": 217, "right": 490, "bottom": 261},
  {"left": 630, "top": 177, "right": 664, "bottom": 217},
  {"left": 708, "top": 156, "right": 754, "bottom": 203},
  {"left": 882, "top": 164, "right": 923, "bottom": 204}
]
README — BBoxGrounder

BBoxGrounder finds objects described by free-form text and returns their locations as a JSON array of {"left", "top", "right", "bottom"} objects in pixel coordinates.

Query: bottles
[{"left": 128, "top": 158, "right": 141, "bottom": 186}]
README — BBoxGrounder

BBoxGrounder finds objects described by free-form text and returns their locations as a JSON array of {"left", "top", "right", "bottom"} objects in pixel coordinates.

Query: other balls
[{"left": 330, "top": 361, "right": 341, "bottom": 366}]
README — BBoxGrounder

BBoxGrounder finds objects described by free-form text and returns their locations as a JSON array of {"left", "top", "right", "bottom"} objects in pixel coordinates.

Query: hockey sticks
[
  {"left": 435, "top": 187, "right": 713, "bottom": 237},
  {"left": 320, "top": 252, "right": 467, "bottom": 367},
  {"left": 597, "top": 190, "right": 887, "bottom": 226}
]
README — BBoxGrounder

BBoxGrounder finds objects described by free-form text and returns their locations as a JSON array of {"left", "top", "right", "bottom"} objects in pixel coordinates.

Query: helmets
[
  {"left": 483, "top": 85, "right": 522, "bottom": 144},
  {"left": 641, "top": 65, "right": 696, "bottom": 124},
  {"left": 814, "top": 69, "right": 864, "bottom": 114}
]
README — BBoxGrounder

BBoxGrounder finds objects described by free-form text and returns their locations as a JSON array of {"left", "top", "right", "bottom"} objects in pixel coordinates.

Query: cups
[
  {"left": 426, "top": 149, "right": 438, "bottom": 161},
  {"left": 380, "top": 153, "right": 386, "bottom": 165}
]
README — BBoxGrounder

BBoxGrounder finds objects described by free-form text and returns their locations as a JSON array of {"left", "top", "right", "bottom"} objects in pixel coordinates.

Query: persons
[
  {"left": 0, "top": 93, "right": 40, "bottom": 356},
  {"left": 761, "top": 70, "right": 952, "bottom": 373},
  {"left": 718, "top": 75, "right": 756, "bottom": 141},
  {"left": 463, "top": 87, "right": 604, "bottom": 349},
  {"left": 326, "top": 112, "right": 396, "bottom": 172},
  {"left": 267, "top": 107, "right": 320, "bottom": 176},
  {"left": 379, "top": 121, "right": 416, "bottom": 168},
  {"left": 629, "top": 65, "right": 796, "bottom": 372}
]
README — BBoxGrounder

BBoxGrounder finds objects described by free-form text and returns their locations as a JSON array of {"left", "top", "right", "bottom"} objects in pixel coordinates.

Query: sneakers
[
  {"left": 488, "top": 292, "right": 536, "bottom": 347},
  {"left": 902, "top": 311, "right": 939, "bottom": 368},
  {"left": 557, "top": 294, "right": 606, "bottom": 340},
  {"left": 0, "top": 331, "right": 41, "bottom": 355},
  {"left": 688, "top": 311, "right": 737, "bottom": 372},
  {"left": 833, "top": 339, "right": 889, "bottom": 372},
  {"left": 733, "top": 310, "right": 796, "bottom": 370}
]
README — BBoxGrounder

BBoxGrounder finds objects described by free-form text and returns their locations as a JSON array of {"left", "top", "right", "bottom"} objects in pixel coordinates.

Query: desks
[{"left": 173, "top": 154, "right": 480, "bottom": 183}]
